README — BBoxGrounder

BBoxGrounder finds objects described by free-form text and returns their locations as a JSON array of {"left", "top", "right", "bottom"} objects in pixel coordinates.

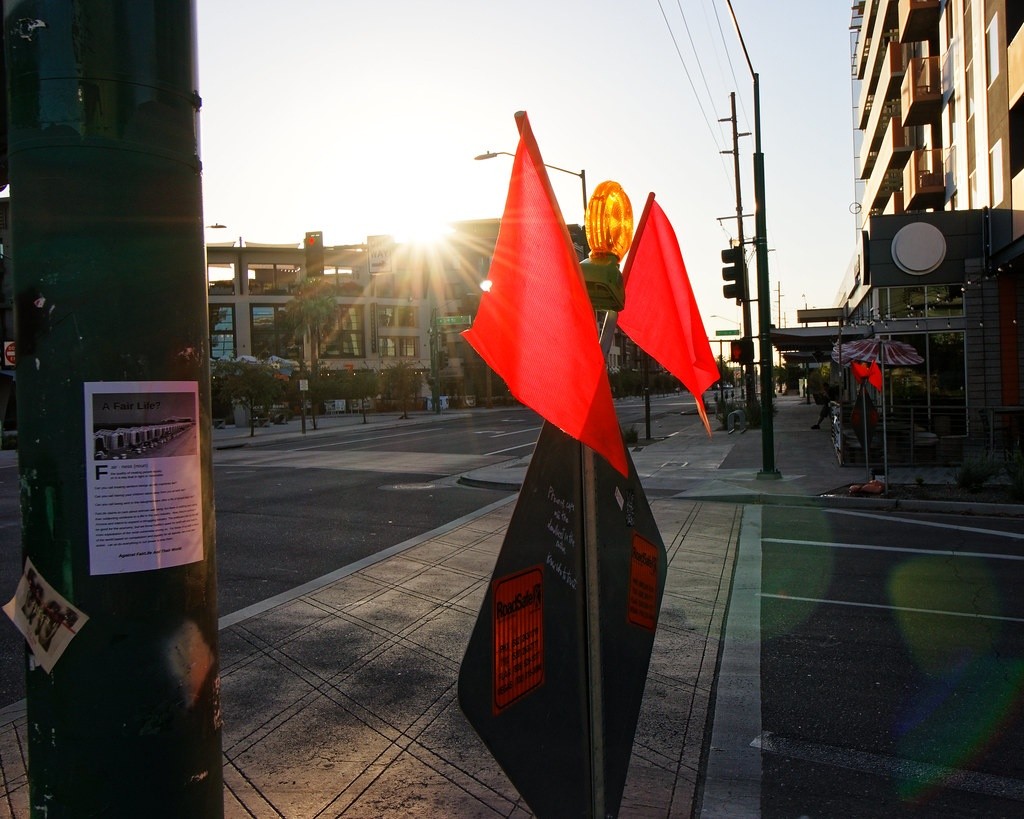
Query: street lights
[
  {"left": 802, "top": 293, "right": 808, "bottom": 327},
  {"left": 475, "top": 149, "right": 589, "bottom": 261},
  {"left": 710, "top": 315, "right": 743, "bottom": 389}
]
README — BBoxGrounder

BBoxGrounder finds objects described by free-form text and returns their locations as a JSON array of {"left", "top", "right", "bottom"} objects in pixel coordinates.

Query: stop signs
[{"left": 4, "top": 341, "right": 15, "bottom": 365}]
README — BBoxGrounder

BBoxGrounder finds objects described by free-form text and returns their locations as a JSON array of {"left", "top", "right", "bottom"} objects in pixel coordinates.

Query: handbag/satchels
[{"left": 851, "top": 384, "right": 878, "bottom": 427}]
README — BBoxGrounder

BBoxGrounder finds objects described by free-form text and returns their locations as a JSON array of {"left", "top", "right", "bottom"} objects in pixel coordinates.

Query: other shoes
[{"left": 812, "top": 425, "right": 820, "bottom": 429}]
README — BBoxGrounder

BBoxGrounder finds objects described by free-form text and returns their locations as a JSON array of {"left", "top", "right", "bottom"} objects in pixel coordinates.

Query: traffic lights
[
  {"left": 721, "top": 246, "right": 744, "bottom": 300},
  {"left": 304, "top": 231, "right": 324, "bottom": 272},
  {"left": 731, "top": 340, "right": 746, "bottom": 363}
]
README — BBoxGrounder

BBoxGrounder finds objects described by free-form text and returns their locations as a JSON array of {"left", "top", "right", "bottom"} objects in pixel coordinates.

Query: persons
[{"left": 811, "top": 382, "right": 837, "bottom": 429}]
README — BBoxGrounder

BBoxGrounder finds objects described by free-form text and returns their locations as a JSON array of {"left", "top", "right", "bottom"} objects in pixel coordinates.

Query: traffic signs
[{"left": 367, "top": 235, "right": 393, "bottom": 274}]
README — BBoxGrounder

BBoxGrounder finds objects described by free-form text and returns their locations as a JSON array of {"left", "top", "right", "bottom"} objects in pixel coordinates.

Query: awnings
[
  {"left": 767, "top": 324, "right": 873, "bottom": 350},
  {"left": 782, "top": 350, "right": 832, "bottom": 363}
]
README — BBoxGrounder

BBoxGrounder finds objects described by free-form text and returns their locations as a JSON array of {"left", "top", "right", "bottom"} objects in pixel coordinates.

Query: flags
[
  {"left": 868, "top": 362, "right": 882, "bottom": 392},
  {"left": 617, "top": 198, "right": 721, "bottom": 435},
  {"left": 460, "top": 126, "right": 628, "bottom": 479},
  {"left": 850, "top": 361, "right": 875, "bottom": 384}
]
